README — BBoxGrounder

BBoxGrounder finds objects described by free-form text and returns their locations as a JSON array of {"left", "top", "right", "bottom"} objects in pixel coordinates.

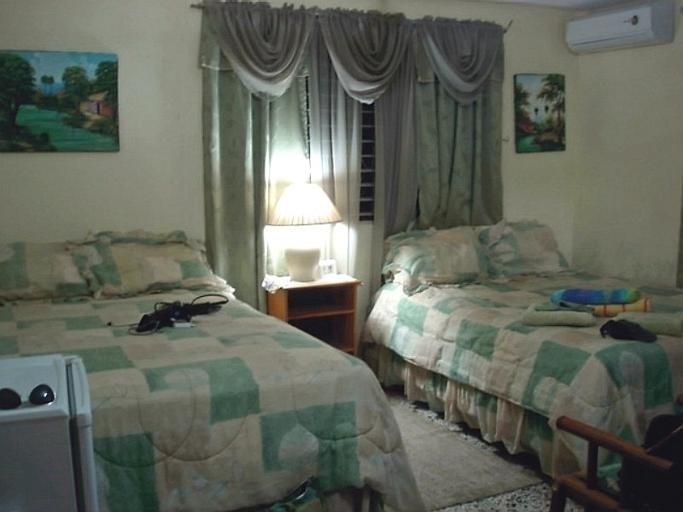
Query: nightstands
[{"left": 264, "top": 270, "right": 362, "bottom": 359}]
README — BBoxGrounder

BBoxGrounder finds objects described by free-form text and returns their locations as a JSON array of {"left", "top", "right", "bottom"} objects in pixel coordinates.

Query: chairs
[{"left": 552, "top": 393, "right": 683, "bottom": 511}]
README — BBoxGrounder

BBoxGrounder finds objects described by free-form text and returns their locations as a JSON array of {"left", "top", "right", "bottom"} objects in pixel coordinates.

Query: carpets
[{"left": 383, "top": 400, "right": 544, "bottom": 512}]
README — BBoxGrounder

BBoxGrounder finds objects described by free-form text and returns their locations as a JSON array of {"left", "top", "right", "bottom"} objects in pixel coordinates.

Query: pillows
[
  {"left": 472, "top": 219, "right": 577, "bottom": 278},
  {"left": 380, "top": 223, "right": 490, "bottom": 297},
  {"left": 0, "top": 241, "right": 93, "bottom": 306},
  {"left": 64, "top": 229, "right": 236, "bottom": 301}
]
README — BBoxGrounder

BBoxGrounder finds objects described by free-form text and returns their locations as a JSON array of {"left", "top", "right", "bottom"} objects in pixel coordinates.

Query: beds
[
  {"left": 359, "top": 219, "right": 683, "bottom": 482},
  {"left": 0, "top": 229, "right": 428, "bottom": 511}
]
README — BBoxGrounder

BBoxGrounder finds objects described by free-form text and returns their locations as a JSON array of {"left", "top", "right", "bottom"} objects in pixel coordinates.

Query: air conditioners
[{"left": 563, "top": 0, "right": 676, "bottom": 56}]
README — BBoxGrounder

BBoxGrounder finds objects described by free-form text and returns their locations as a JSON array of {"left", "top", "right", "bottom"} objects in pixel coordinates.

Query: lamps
[{"left": 266, "top": 183, "right": 343, "bottom": 282}]
